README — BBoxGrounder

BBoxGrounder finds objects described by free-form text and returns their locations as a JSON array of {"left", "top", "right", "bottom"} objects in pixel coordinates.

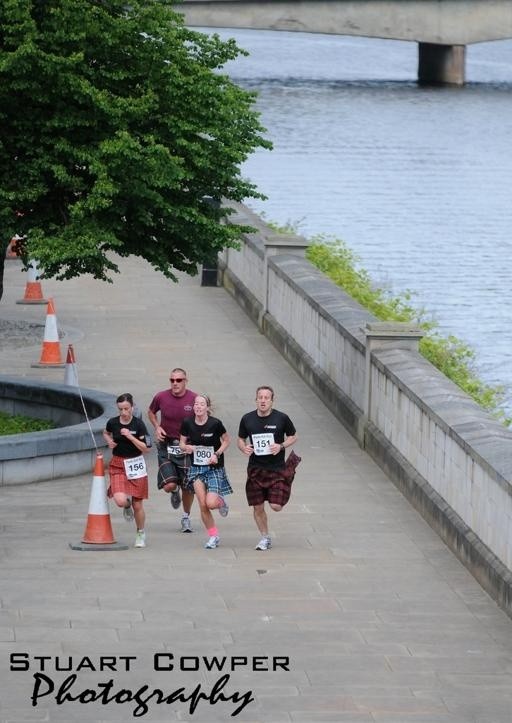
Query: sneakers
[
  {"left": 218, "top": 496, "right": 229, "bottom": 517},
  {"left": 254, "top": 536, "right": 273, "bottom": 551},
  {"left": 122, "top": 499, "right": 134, "bottom": 522},
  {"left": 170, "top": 486, "right": 181, "bottom": 510},
  {"left": 205, "top": 535, "right": 221, "bottom": 549},
  {"left": 134, "top": 529, "right": 147, "bottom": 549},
  {"left": 181, "top": 517, "right": 193, "bottom": 533}
]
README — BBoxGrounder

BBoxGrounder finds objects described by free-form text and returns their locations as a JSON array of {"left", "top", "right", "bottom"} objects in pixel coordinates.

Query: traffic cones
[
  {"left": 15, "top": 259, "right": 53, "bottom": 305},
  {"left": 5, "top": 210, "right": 33, "bottom": 260},
  {"left": 62, "top": 341, "right": 80, "bottom": 388},
  {"left": 69, "top": 452, "right": 130, "bottom": 552},
  {"left": 30, "top": 297, "right": 71, "bottom": 369}
]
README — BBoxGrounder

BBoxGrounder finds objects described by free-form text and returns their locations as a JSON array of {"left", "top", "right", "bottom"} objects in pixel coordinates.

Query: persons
[
  {"left": 178, "top": 393, "right": 234, "bottom": 549},
  {"left": 237, "top": 387, "right": 298, "bottom": 551},
  {"left": 147, "top": 368, "right": 199, "bottom": 533},
  {"left": 102, "top": 393, "right": 153, "bottom": 548}
]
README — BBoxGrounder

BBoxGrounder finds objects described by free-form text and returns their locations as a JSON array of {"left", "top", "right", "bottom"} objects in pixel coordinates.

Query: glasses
[{"left": 170, "top": 378, "right": 185, "bottom": 383}]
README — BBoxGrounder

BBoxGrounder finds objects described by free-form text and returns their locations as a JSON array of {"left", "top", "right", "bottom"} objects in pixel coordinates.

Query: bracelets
[
  {"left": 214, "top": 452, "right": 219, "bottom": 457},
  {"left": 281, "top": 443, "right": 285, "bottom": 451}
]
